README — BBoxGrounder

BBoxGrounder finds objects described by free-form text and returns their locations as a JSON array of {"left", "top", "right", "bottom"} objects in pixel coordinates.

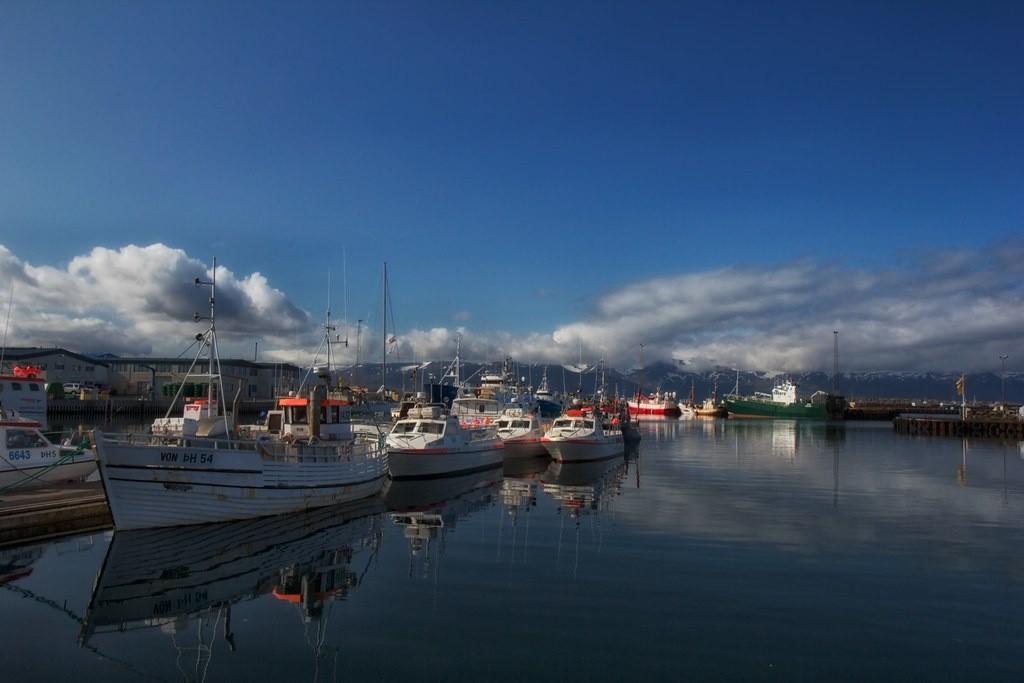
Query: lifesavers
[{"left": 474, "top": 384, "right": 481, "bottom": 396}]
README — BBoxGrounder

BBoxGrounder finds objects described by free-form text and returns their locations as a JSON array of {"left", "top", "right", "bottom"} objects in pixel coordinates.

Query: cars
[{"left": 83, "top": 385, "right": 99, "bottom": 395}]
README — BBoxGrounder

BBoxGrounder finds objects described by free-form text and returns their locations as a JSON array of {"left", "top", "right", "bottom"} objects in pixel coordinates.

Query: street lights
[{"left": 996, "top": 353, "right": 1009, "bottom": 407}]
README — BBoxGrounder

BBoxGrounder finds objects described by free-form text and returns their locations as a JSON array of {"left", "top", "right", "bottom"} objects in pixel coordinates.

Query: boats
[
  {"left": 503, "top": 454, "right": 552, "bottom": 480},
  {"left": 383, "top": 462, "right": 505, "bottom": 579},
  {"left": 73, "top": 493, "right": 394, "bottom": 682},
  {"left": 537, "top": 452, "right": 625, "bottom": 521},
  {"left": 0, "top": 258, "right": 845, "bottom": 534}
]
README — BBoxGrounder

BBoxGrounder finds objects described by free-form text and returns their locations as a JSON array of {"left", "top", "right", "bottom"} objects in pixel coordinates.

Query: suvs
[{"left": 62, "top": 382, "right": 83, "bottom": 396}]
